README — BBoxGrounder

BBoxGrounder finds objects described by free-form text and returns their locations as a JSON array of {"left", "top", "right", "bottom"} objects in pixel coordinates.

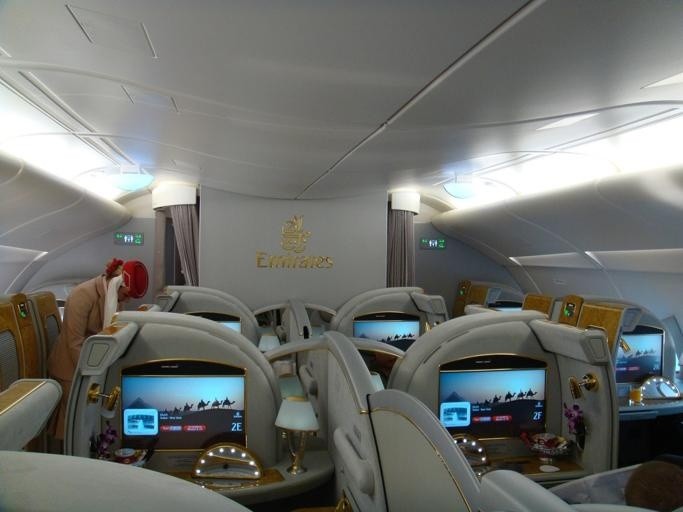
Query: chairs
[
  {"left": 569, "top": 299, "right": 681, "bottom": 464},
  {"left": 0, "top": 377, "right": 255, "bottom": 511},
  {"left": 330, "top": 286, "right": 448, "bottom": 353},
  {"left": 388, "top": 307, "right": 621, "bottom": 490},
  {"left": 161, "top": 283, "right": 260, "bottom": 349}
]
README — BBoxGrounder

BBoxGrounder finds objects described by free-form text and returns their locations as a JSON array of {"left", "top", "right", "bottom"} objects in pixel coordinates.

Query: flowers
[{"left": 562, "top": 401, "right": 583, "bottom": 435}]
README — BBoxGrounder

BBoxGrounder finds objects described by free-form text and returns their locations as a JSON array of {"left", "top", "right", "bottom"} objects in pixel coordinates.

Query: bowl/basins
[{"left": 528, "top": 432, "right": 570, "bottom": 456}]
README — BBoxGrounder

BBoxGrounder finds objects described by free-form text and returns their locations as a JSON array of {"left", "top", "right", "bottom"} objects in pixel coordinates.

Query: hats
[{"left": 122, "top": 260, "right": 149, "bottom": 299}]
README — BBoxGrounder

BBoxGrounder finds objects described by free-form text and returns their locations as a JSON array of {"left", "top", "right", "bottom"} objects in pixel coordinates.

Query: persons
[{"left": 46, "top": 258, "right": 149, "bottom": 454}]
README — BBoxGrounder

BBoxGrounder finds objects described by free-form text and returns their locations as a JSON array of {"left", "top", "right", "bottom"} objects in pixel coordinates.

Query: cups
[
  {"left": 628, "top": 384, "right": 643, "bottom": 404},
  {"left": 113, "top": 448, "right": 136, "bottom": 463}
]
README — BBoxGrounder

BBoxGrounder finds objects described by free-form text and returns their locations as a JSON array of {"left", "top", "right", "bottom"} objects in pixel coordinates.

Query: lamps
[
  {"left": 273, "top": 397, "right": 322, "bottom": 475},
  {"left": 370, "top": 372, "right": 386, "bottom": 391},
  {"left": 87, "top": 382, "right": 121, "bottom": 418},
  {"left": 257, "top": 334, "right": 281, "bottom": 353}
]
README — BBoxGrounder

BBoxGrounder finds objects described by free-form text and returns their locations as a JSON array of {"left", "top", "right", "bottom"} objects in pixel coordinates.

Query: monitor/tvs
[
  {"left": 353, "top": 320, "right": 421, "bottom": 351},
  {"left": 121, "top": 374, "right": 246, "bottom": 451},
  {"left": 616, "top": 334, "right": 663, "bottom": 382},
  {"left": 439, "top": 369, "right": 547, "bottom": 442}
]
